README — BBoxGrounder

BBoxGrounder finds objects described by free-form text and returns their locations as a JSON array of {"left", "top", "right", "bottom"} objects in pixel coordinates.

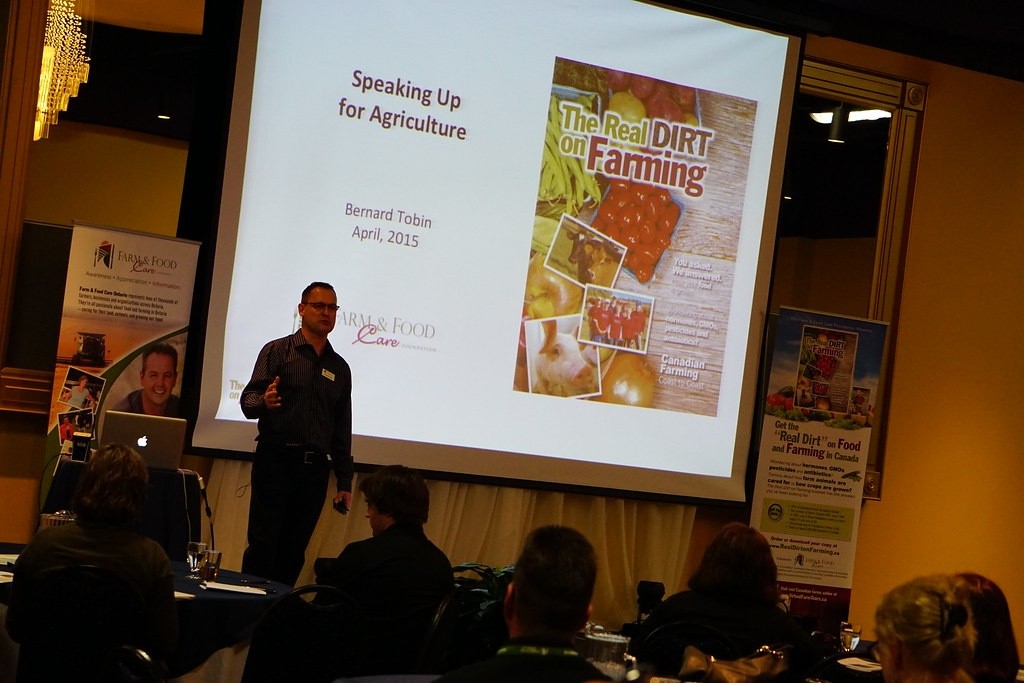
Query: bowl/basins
[{"left": 551, "top": 83, "right": 601, "bottom": 119}]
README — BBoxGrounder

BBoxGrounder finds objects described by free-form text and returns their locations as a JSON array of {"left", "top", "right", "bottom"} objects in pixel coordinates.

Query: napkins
[{"left": 837, "top": 657, "right": 882, "bottom": 672}]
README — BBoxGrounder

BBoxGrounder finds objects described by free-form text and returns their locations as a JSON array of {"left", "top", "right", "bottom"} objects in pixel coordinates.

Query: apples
[{"left": 525, "top": 253, "right": 583, "bottom": 319}]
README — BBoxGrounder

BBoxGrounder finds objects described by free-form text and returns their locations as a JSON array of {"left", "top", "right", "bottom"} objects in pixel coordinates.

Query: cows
[{"left": 566, "top": 229, "right": 622, "bottom": 287}]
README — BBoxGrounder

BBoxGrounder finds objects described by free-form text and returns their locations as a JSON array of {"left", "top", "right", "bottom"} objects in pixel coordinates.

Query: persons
[
  {"left": 60, "top": 414, "right": 88, "bottom": 443},
  {"left": 433, "top": 525, "right": 617, "bottom": 683},
  {"left": 113, "top": 345, "right": 183, "bottom": 418},
  {"left": 628, "top": 524, "right": 812, "bottom": 673},
  {"left": 586, "top": 294, "right": 646, "bottom": 351},
  {"left": 238, "top": 282, "right": 355, "bottom": 587},
  {"left": 3, "top": 441, "right": 182, "bottom": 682},
  {"left": 870, "top": 571, "right": 1024, "bottom": 683},
  {"left": 294, "top": 461, "right": 454, "bottom": 682},
  {"left": 64, "top": 375, "right": 94, "bottom": 408}
]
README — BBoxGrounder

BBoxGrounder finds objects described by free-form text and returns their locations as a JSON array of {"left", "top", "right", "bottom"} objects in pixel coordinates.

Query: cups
[
  {"left": 205, "top": 550, "right": 222, "bottom": 582},
  {"left": 586, "top": 633, "right": 636, "bottom": 681},
  {"left": 72, "top": 432, "right": 91, "bottom": 462}
]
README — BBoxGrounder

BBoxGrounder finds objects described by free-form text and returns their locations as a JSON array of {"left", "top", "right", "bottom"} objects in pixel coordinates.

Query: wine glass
[
  {"left": 185, "top": 541, "right": 207, "bottom": 579},
  {"left": 840, "top": 621, "right": 862, "bottom": 654}
]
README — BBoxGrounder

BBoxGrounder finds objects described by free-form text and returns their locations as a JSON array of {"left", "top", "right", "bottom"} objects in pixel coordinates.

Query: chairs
[
  {"left": 10, "top": 564, "right": 160, "bottom": 683},
  {"left": 634, "top": 621, "right": 740, "bottom": 682},
  {"left": 241, "top": 585, "right": 375, "bottom": 683}
]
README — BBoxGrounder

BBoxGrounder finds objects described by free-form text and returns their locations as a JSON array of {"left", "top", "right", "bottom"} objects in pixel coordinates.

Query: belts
[{"left": 301, "top": 451, "right": 321, "bottom": 463}]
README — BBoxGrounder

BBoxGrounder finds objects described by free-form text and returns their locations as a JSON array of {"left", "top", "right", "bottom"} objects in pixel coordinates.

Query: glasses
[{"left": 304, "top": 301, "right": 339, "bottom": 312}]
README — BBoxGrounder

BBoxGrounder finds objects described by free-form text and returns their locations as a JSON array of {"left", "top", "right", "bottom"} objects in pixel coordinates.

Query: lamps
[{"left": 827, "top": 101, "right": 851, "bottom": 144}]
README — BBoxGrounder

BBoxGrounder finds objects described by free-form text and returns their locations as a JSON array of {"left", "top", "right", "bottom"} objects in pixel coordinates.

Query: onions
[{"left": 594, "top": 354, "right": 657, "bottom": 407}]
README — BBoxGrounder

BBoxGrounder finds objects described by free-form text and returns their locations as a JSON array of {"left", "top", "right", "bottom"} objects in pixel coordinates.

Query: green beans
[{"left": 538, "top": 93, "right": 604, "bottom": 217}]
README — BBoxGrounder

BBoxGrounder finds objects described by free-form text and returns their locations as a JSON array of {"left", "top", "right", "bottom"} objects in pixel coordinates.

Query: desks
[
  {"left": 816, "top": 641, "right": 883, "bottom": 683},
  {"left": 0, "top": 552, "right": 306, "bottom": 683},
  {"left": 33, "top": 457, "right": 201, "bottom": 562}
]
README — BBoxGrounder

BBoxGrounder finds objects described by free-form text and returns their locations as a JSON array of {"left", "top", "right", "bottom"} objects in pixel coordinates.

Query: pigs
[{"left": 533, "top": 319, "right": 595, "bottom": 395}]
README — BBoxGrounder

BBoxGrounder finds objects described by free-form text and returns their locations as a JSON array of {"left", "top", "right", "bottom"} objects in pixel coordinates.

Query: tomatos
[
  {"left": 590, "top": 178, "right": 680, "bottom": 284},
  {"left": 607, "top": 70, "right": 697, "bottom": 127}
]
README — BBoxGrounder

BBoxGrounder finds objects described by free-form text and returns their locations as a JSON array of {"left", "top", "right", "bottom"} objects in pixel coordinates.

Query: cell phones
[{"left": 332, "top": 499, "right": 349, "bottom": 515}]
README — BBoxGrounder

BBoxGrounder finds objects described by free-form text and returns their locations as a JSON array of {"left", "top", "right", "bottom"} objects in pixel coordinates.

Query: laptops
[{"left": 100, "top": 410, "right": 187, "bottom": 473}]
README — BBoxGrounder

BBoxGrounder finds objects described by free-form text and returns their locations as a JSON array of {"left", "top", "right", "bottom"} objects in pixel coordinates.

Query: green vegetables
[{"left": 552, "top": 57, "right": 612, "bottom": 97}]
